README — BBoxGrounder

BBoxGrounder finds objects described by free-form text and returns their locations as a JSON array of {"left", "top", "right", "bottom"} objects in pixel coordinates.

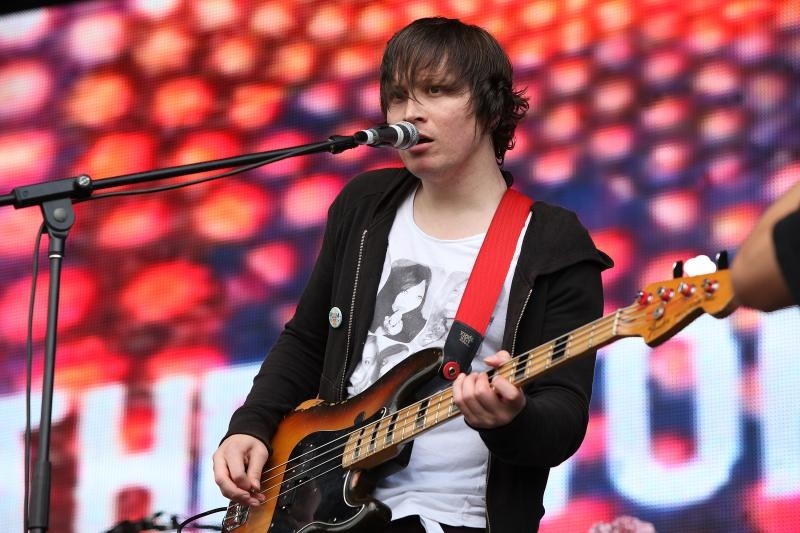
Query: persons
[
  {"left": 213, "top": 17, "right": 615, "bottom": 533},
  {"left": 729, "top": 180, "right": 800, "bottom": 313}
]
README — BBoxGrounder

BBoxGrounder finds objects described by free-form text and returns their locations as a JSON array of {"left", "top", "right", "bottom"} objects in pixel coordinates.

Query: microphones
[{"left": 352, "top": 118, "right": 419, "bottom": 151}]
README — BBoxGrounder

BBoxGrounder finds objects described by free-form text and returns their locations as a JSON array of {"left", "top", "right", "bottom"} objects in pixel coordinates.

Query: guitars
[{"left": 221, "top": 250, "right": 740, "bottom": 533}]
